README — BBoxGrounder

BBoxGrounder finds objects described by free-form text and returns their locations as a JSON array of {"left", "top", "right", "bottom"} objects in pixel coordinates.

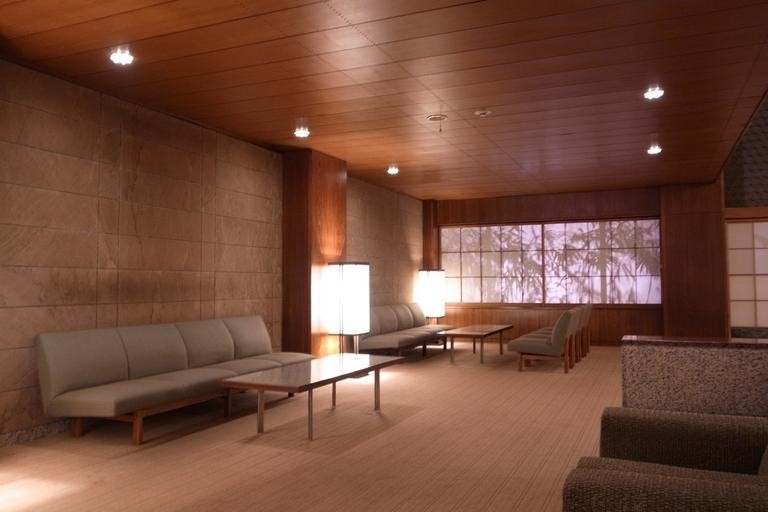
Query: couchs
[
  {"left": 34, "top": 314, "right": 316, "bottom": 446},
  {"left": 359, "top": 303, "right": 455, "bottom": 356}
]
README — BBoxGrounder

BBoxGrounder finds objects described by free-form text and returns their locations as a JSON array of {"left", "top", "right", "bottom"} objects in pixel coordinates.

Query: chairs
[
  {"left": 507, "top": 304, "right": 592, "bottom": 373},
  {"left": 563, "top": 407, "right": 768, "bottom": 512}
]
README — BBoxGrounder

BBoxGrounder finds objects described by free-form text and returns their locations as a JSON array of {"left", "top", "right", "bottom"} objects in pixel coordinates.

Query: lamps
[
  {"left": 327, "top": 262, "right": 371, "bottom": 379},
  {"left": 419, "top": 269, "right": 446, "bottom": 345}
]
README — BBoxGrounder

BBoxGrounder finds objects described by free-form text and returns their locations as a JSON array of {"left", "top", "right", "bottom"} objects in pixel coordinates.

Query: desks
[
  {"left": 219, "top": 353, "right": 407, "bottom": 440},
  {"left": 437, "top": 324, "right": 513, "bottom": 364}
]
